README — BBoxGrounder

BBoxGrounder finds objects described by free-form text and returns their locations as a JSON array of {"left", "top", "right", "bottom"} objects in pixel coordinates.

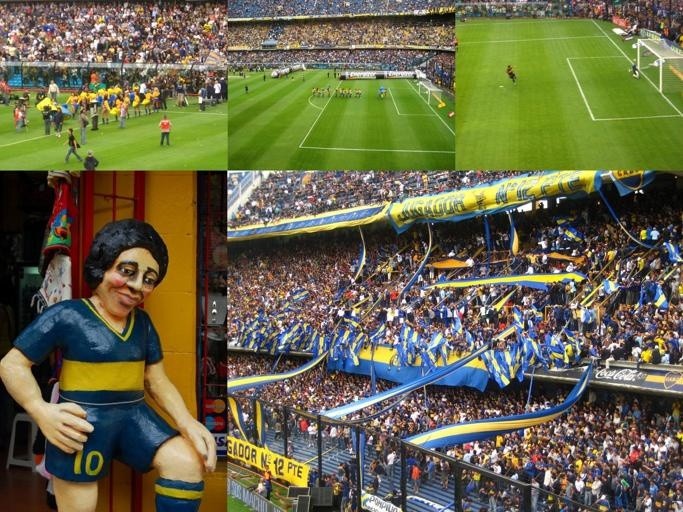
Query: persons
[
  {"left": 507, "top": 64, "right": 517, "bottom": 85},
  {"left": 228, "top": 0, "right": 455, "bottom": 102},
  {"left": 455, "top": 0, "right": 683, "bottom": 50},
  {"left": 631, "top": 63, "right": 639, "bottom": 79},
  {"left": 227, "top": 169, "right": 682, "bottom": 512},
  {"left": 0, "top": 0, "right": 229, "bottom": 171},
  {"left": 0, "top": 217, "right": 219, "bottom": 510}
]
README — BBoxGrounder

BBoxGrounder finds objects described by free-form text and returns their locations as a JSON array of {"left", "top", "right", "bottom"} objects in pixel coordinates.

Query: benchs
[{"left": 256, "top": 420, "right": 505, "bottom": 512}]
